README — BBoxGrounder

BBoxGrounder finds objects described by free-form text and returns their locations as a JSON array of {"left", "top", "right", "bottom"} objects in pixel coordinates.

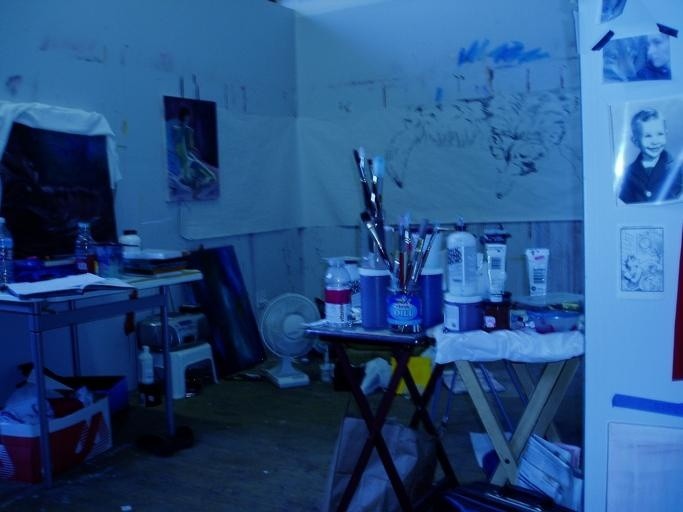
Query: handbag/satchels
[{"left": 321, "top": 415, "right": 447, "bottom": 512}]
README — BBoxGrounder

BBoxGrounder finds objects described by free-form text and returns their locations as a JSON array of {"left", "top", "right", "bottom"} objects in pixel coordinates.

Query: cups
[
  {"left": 358, "top": 267, "right": 392, "bottom": 330},
  {"left": 418, "top": 268, "right": 444, "bottom": 329}
]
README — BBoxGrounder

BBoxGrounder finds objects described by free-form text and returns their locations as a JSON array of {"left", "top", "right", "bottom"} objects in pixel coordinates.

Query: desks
[
  {"left": 0, "top": 266, "right": 205, "bottom": 489},
  {"left": 302, "top": 320, "right": 458, "bottom": 510},
  {"left": 424, "top": 324, "right": 584, "bottom": 487}
]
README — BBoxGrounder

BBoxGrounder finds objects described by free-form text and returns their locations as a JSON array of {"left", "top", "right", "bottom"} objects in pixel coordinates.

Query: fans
[{"left": 258, "top": 293, "right": 321, "bottom": 389}]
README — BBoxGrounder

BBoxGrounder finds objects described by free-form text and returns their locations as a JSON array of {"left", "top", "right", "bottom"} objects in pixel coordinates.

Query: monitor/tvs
[{"left": 0, "top": 102, "right": 119, "bottom": 260}]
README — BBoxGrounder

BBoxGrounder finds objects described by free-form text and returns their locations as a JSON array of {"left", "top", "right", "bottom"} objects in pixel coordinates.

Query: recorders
[{"left": 137, "top": 310, "right": 209, "bottom": 352}]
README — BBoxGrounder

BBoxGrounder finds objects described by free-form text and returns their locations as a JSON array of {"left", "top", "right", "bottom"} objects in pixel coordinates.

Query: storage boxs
[
  {"left": 0, "top": 396, "right": 114, "bottom": 482},
  {"left": 64, "top": 375, "right": 128, "bottom": 414}
]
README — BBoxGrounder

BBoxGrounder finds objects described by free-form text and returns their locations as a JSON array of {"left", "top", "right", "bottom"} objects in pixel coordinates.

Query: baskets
[{"left": 441, "top": 481, "right": 572, "bottom": 512}]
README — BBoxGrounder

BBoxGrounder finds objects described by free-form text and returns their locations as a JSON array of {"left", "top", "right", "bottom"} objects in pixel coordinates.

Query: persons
[
  {"left": 618, "top": 108, "right": 682, "bottom": 203},
  {"left": 637, "top": 36, "right": 671, "bottom": 80}
]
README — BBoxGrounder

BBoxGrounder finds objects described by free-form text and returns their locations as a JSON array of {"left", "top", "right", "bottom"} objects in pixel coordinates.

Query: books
[{"left": 6, "top": 273, "right": 134, "bottom": 298}]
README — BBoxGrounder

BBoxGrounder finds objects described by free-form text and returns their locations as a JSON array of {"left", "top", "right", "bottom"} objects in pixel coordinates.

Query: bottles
[
  {"left": 0, "top": 218, "right": 14, "bottom": 291},
  {"left": 117, "top": 229, "right": 141, "bottom": 258},
  {"left": 483, "top": 296, "right": 504, "bottom": 331},
  {"left": 137, "top": 345, "right": 158, "bottom": 407},
  {"left": 74, "top": 221, "right": 98, "bottom": 274},
  {"left": 444, "top": 222, "right": 480, "bottom": 296},
  {"left": 323, "top": 258, "right": 353, "bottom": 329},
  {"left": 503, "top": 292, "right": 511, "bottom": 330},
  {"left": 384, "top": 280, "right": 425, "bottom": 334},
  {"left": 343, "top": 256, "right": 361, "bottom": 306}
]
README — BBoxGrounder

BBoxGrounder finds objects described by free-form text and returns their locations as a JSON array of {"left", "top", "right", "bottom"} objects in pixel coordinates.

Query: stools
[{"left": 149, "top": 342, "right": 218, "bottom": 400}]
